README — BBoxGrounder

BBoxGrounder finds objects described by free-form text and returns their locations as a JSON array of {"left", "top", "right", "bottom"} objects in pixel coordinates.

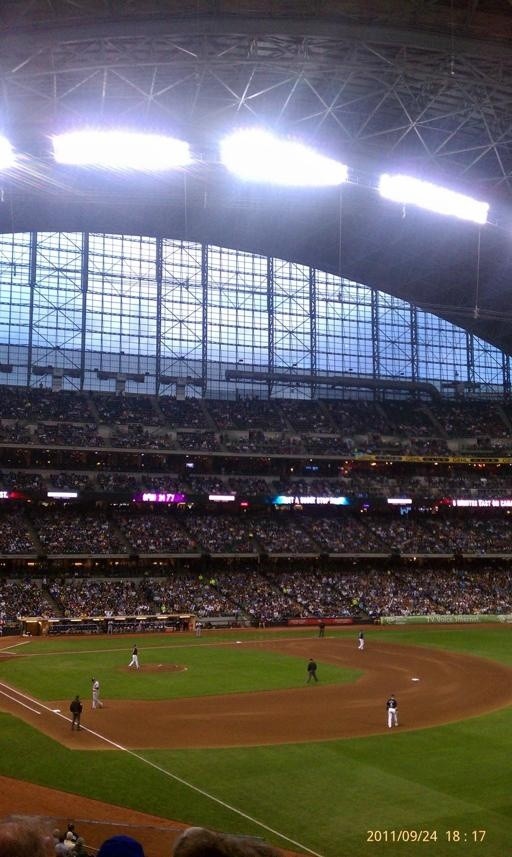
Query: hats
[{"left": 99, "top": 836, "right": 144, "bottom": 857}]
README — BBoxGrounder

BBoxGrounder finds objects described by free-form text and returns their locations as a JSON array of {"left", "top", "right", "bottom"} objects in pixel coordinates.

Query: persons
[
  {"left": 91, "top": 678, "right": 104, "bottom": 710},
  {"left": 70, "top": 695, "right": 83, "bottom": 731},
  {"left": 196, "top": 621, "right": 202, "bottom": 637},
  {"left": 357, "top": 628, "right": 365, "bottom": 651},
  {"left": 386, "top": 694, "right": 399, "bottom": 729},
  {"left": 0, "top": 814, "right": 285, "bottom": 857},
  {"left": 306, "top": 659, "right": 318, "bottom": 683},
  {"left": 318, "top": 619, "right": 325, "bottom": 638},
  {"left": 128, "top": 644, "right": 140, "bottom": 671}
]
